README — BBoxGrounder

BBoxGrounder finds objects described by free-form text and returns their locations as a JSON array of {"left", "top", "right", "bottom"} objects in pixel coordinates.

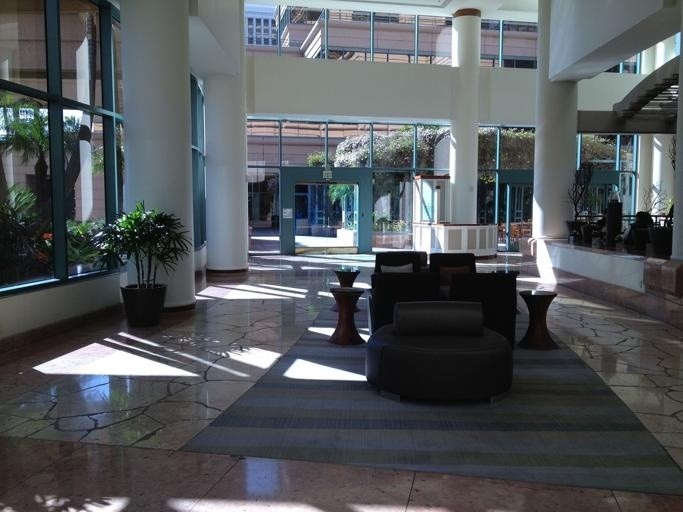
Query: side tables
[
  {"left": 497, "top": 269, "right": 560, "bottom": 351},
  {"left": 328, "top": 267, "right": 366, "bottom": 346}
]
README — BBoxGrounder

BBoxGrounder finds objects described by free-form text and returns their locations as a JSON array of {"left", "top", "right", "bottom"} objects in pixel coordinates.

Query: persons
[{"left": 596, "top": 208, "right": 608, "bottom": 226}]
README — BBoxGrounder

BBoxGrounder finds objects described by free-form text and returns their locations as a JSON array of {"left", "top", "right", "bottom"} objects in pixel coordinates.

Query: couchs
[{"left": 364, "top": 301, "right": 513, "bottom": 404}]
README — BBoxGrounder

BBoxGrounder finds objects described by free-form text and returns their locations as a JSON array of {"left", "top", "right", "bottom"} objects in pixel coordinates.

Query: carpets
[{"left": 175, "top": 297, "right": 683, "bottom": 497}]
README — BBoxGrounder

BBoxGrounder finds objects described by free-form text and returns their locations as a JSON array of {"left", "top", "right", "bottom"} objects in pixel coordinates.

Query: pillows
[
  {"left": 439, "top": 266, "right": 469, "bottom": 273},
  {"left": 380, "top": 263, "right": 413, "bottom": 273}
]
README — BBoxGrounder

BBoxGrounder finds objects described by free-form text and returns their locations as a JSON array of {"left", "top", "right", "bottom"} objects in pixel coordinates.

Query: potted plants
[{"left": 89, "top": 199, "right": 194, "bottom": 328}]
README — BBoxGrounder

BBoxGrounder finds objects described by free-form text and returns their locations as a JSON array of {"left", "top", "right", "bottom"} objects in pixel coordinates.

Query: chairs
[
  {"left": 566, "top": 221, "right": 673, "bottom": 260},
  {"left": 366, "top": 251, "right": 519, "bottom": 340}
]
